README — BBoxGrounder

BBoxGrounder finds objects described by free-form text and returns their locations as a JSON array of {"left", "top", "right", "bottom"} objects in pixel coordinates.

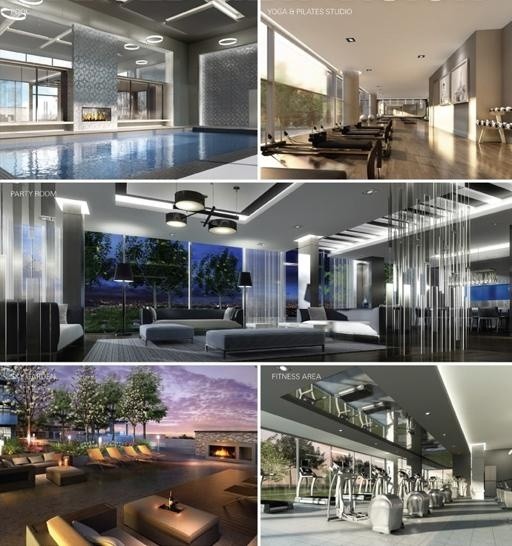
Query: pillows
[
  {"left": 10, "top": 455, "right": 30, "bottom": 467},
  {"left": 146, "top": 306, "right": 158, "bottom": 320},
  {"left": 71, "top": 517, "right": 101, "bottom": 545},
  {"left": 45, "top": 452, "right": 62, "bottom": 460},
  {"left": 94, "top": 534, "right": 128, "bottom": 546},
  {"left": 306, "top": 305, "right": 328, "bottom": 322},
  {"left": 223, "top": 307, "right": 235, "bottom": 321},
  {"left": 29, "top": 454, "right": 42, "bottom": 462},
  {"left": 55, "top": 303, "right": 69, "bottom": 322}
]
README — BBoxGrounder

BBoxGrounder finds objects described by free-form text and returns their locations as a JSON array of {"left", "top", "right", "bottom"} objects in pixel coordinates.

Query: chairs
[
  {"left": 409, "top": 299, "right": 510, "bottom": 334},
  {"left": 217, "top": 483, "right": 258, "bottom": 512},
  {"left": 84, "top": 436, "right": 167, "bottom": 473},
  {"left": 23, "top": 503, "right": 181, "bottom": 545},
  {"left": 243, "top": 474, "right": 255, "bottom": 485}
]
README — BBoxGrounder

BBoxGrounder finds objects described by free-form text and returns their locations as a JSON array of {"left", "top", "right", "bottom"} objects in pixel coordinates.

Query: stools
[
  {"left": 42, "top": 462, "right": 84, "bottom": 486},
  {"left": 138, "top": 320, "right": 193, "bottom": 344}
]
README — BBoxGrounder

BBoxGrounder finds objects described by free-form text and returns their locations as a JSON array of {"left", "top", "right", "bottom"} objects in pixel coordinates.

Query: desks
[
  {"left": 119, "top": 495, "right": 224, "bottom": 546},
  {"left": 261, "top": 108, "right": 394, "bottom": 179}
]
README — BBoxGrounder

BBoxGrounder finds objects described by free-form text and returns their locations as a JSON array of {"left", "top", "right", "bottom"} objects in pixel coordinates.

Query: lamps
[
  {"left": 447, "top": 247, "right": 497, "bottom": 287},
  {"left": 159, "top": 183, "right": 244, "bottom": 238},
  {"left": 237, "top": 270, "right": 253, "bottom": 327}
]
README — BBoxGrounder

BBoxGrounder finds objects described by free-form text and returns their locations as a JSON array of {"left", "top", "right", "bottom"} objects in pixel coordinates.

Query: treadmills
[
  {"left": 260, "top": 469, "right": 293, "bottom": 513},
  {"left": 334, "top": 462, "right": 372, "bottom": 500},
  {"left": 295, "top": 467, "right": 335, "bottom": 504}
]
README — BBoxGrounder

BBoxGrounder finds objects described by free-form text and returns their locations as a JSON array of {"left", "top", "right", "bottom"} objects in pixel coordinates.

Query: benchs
[{"left": 204, "top": 324, "right": 329, "bottom": 357}]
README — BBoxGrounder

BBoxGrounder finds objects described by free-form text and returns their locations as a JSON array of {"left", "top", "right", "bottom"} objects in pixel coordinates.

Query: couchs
[
  {"left": 1, "top": 297, "right": 87, "bottom": 365},
  {"left": 292, "top": 303, "right": 407, "bottom": 347},
  {"left": 0, "top": 449, "right": 73, "bottom": 493},
  {"left": 138, "top": 306, "right": 245, "bottom": 338}
]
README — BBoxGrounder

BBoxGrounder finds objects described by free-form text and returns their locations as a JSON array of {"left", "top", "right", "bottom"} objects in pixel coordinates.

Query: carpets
[{"left": 83, "top": 333, "right": 393, "bottom": 362}]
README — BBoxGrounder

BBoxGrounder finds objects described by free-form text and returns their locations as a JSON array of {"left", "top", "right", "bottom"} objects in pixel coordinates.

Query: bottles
[{"left": 168, "top": 489, "right": 174, "bottom": 509}]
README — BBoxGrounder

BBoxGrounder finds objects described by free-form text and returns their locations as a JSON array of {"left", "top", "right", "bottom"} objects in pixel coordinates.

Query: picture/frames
[
  {"left": 448, "top": 58, "right": 472, "bottom": 107},
  {"left": 437, "top": 71, "right": 452, "bottom": 107}
]
